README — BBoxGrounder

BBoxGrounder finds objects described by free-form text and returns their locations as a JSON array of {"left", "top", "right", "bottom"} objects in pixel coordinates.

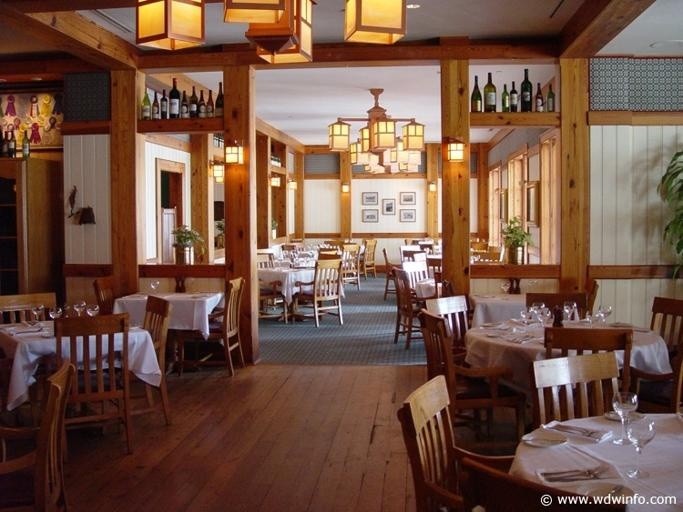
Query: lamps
[
  {"left": 208, "top": 137, "right": 299, "bottom": 193},
  {"left": 348, "top": 137, "right": 421, "bottom": 174},
  {"left": 327, "top": 89, "right": 425, "bottom": 152},
  {"left": 134, "top": 1, "right": 408, "bottom": 63},
  {"left": 341, "top": 182, "right": 349, "bottom": 193},
  {"left": 446, "top": 137, "right": 467, "bottom": 163},
  {"left": 429, "top": 181, "right": 436, "bottom": 191}
]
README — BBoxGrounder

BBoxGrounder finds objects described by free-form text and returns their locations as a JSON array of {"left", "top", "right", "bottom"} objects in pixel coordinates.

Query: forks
[{"left": 541, "top": 464, "right": 606, "bottom": 482}]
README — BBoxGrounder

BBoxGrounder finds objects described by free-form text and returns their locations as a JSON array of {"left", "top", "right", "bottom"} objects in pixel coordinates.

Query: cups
[
  {"left": 48, "top": 305, "right": 62, "bottom": 320},
  {"left": 83, "top": 303, "right": 99, "bottom": 316}
]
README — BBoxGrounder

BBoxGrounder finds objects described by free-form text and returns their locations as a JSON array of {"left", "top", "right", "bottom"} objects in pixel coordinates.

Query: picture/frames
[
  {"left": 362, "top": 192, "right": 378, "bottom": 204},
  {"left": 400, "top": 192, "right": 417, "bottom": 205},
  {"left": 400, "top": 209, "right": 416, "bottom": 222},
  {"left": 382, "top": 199, "right": 396, "bottom": 214},
  {"left": 362, "top": 209, "right": 379, "bottom": 223}
]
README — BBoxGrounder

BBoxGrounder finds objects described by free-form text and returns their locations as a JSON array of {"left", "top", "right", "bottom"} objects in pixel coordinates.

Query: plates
[
  {"left": 574, "top": 480, "right": 635, "bottom": 505},
  {"left": 481, "top": 322, "right": 545, "bottom": 349},
  {"left": 522, "top": 430, "right": 568, "bottom": 448},
  {"left": 603, "top": 410, "right": 644, "bottom": 421}
]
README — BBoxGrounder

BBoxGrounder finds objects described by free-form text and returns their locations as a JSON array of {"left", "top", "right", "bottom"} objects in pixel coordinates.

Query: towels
[{"left": 533, "top": 461, "right": 626, "bottom": 486}]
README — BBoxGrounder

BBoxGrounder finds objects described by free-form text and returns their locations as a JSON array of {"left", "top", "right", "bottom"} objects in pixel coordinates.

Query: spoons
[{"left": 603, "top": 484, "right": 623, "bottom": 499}]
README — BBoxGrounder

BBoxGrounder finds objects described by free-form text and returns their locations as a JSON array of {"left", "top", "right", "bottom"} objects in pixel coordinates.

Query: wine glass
[
  {"left": 72, "top": 298, "right": 85, "bottom": 316},
  {"left": 30, "top": 302, "right": 45, "bottom": 321},
  {"left": 516, "top": 300, "right": 614, "bottom": 330},
  {"left": 149, "top": 279, "right": 160, "bottom": 294},
  {"left": 624, "top": 416, "right": 656, "bottom": 479},
  {"left": 610, "top": 391, "right": 638, "bottom": 445}
]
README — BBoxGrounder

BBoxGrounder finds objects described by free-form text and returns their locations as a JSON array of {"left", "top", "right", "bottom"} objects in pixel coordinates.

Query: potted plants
[
  {"left": 214, "top": 219, "right": 226, "bottom": 248},
  {"left": 171, "top": 226, "right": 199, "bottom": 263},
  {"left": 499, "top": 217, "right": 531, "bottom": 262}
]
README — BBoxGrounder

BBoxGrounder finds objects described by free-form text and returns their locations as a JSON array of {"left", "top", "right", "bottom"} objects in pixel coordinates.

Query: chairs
[
  {"left": 177, "top": 277, "right": 247, "bottom": 377},
  {"left": 257, "top": 237, "right": 377, "bottom": 329},
  {"left": 399, "top": 373, "right": 517, "bottom": 511},
  {"left": 100, "top": 294, "right": 174, "bottom": 435},
  {"left": 0, "top": 358, "right": 74, "bottom": 512},
  {"left": 0, "top": 292, "right": 58, "bottom": 324},
  {"left": 418, "top": 291, "right": 682, "bottom": 441},
  {"left": 92, "top": 278, "right": 116, "bottom": 310},
  {"left": 382, "top": 235, "right": 498, "bottom": 349},
  {"left": 52, "top": 312, "right": 134, "bottom": 451}
]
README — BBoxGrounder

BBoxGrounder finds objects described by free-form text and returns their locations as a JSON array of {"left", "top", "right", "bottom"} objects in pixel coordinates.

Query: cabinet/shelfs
[{"left": 0, "top": 159, "right": 65, "bottom": 294}]
